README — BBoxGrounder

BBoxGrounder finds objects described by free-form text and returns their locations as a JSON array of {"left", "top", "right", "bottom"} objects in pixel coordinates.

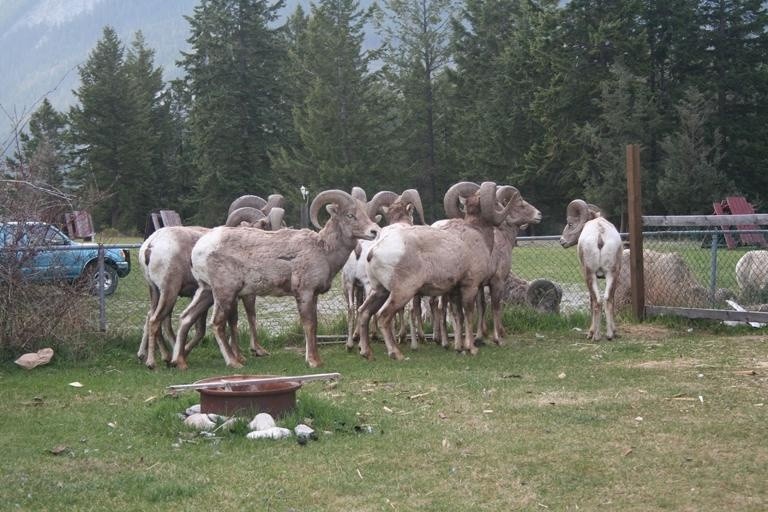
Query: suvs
[{"left": 0, "top": 222, "right": 132, "bottom": 297}]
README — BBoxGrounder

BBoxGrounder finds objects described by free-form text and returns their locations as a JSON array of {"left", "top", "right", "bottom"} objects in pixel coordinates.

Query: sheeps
[
  {"left": 733, "top": 250, "right": 768, "bottom": 302},
  {"left": 136, "top": 186, "right": 378, "bottom": 368},
  {"left": 615, "top": 244, "right": 737, "bottom": 310},
  {"left": 557, "top": 199, "right": 624, "bottom": 344},
  {"left": 339, "top": 183, "right": 543, "bottom": 363}
]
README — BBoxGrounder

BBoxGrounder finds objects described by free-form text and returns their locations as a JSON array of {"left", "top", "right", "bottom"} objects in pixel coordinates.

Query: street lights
[{"left": 299, "top": 186, "right": 310, "bottom": 228}]
